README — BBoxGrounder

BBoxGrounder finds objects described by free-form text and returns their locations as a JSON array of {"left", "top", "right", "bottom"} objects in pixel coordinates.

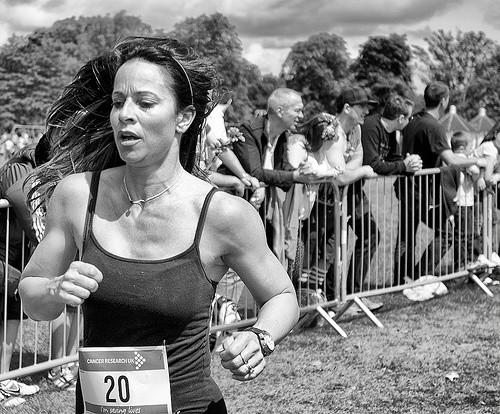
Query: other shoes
[
  {"left": 327, "top": 311, "right": 335, "bottom": 319},
  {"left": 456, "top": 261, "right": 479, "bottom": 271},
  {"left": 479, "top": 254, "right": 497, "bottom": 268},
  {"left": 476, "top": 261, "right": 488, "bottom": 268},
  {"left": 351, "top": 298, "right": 383, "bottom": 313},
  {"left": 491, "top": 252, "right": 500, "bottom": 266}
]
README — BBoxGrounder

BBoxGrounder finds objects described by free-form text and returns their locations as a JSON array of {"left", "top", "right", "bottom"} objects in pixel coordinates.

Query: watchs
[{"left": 240, "top": 326, "right": 276, "bottom": 357}]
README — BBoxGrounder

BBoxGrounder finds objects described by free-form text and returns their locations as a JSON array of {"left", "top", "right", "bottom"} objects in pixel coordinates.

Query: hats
[{"left": 337, "top": 87, "right": 378, "bottom": 109}]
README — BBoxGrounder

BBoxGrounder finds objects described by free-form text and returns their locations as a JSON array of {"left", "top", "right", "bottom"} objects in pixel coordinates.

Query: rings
[
  {"left": 246, "top": 362, "right": 255, "bottom": 374},
  {"left": 239, "top": 353, "right": 248, "bottom": 364}
]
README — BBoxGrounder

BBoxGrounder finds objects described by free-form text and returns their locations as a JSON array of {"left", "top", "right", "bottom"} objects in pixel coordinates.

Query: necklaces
[{"left": 122, "top": 167, "right": 185, "bottom": 206}]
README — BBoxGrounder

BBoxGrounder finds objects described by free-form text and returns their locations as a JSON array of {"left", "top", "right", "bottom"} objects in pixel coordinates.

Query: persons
[
  {"left": 30, "top": 151, "right": 93, "bottom": 391},
  {"left": 0, "top": 120, "right": 80, "bottom": 400},
  {"left": 194, "top": 80, "right": 500, "bottom": 359},
  {"left": 16, "top": 35, "right": 301, "bottom": 414}
]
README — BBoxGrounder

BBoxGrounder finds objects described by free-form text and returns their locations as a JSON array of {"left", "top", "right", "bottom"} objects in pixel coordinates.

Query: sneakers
[
  {"left": 0, "top": 379, "right": 40, "bottom": 397},
  {"left": 0, "top": 391, "right": 28, "bottom": 408},
  {"left": 48, "top": 370, "right": 76, "bottom": 392}
]
